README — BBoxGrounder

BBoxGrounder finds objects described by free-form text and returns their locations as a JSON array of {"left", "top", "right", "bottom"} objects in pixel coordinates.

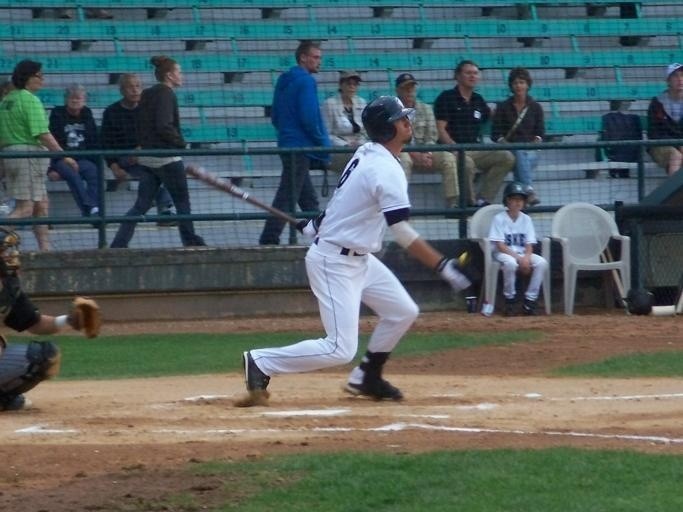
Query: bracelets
[{"left": 54, "top": 315, "right": 70, "bottom": 328}]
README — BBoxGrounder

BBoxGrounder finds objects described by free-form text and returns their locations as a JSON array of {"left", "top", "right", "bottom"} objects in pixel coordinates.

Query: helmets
[
  {"left": 362, "top": 95, "right": 416, "bottom": 143},
  {"left": 621, "top": 289, "right": 655, "bottom": 314},
  {"left": 502, "top": 182, "right": 525, "bottom": 203},
  {"left": 0, "top": 228, "right": 21, "bottom": 276}
]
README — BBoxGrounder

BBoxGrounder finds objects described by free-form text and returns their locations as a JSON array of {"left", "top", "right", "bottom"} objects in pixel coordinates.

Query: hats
[
  {"left": 340, "top": 71, "right": 361, "bottom": 82},
  {"left": 666, "top": 63, "right": 683, "bottom": 79},
  {"left": 396, "top": 74, "right": 417, "bottom": 88}
]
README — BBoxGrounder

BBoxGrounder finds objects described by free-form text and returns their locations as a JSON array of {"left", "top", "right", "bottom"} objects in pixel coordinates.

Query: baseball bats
[{"left": 186, "top": 161, "right": 300, "bottom": 229}]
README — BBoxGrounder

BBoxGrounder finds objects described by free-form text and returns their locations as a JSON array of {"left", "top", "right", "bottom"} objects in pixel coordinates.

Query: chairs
[
  {"left": 551, "top": 202, "right": 633, "bottom": 319},
  {"left": 467, "top": 203, "right": 553, "bottom": 316}
]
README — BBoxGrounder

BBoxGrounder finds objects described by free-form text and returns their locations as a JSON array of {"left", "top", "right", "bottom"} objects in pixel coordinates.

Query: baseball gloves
[{"left": 68, "top": 294, "right": 100, "bottom": 338}]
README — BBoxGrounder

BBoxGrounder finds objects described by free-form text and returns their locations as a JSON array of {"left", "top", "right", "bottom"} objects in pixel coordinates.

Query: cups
[{"left": 463, "top": 296, "right": 476, "bottom": 313}]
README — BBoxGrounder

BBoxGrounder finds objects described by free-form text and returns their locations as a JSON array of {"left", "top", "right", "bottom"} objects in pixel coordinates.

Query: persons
[
  {"left": 102, "top": 74, "right": 178, "bottom": 226},
  {"left": 646, "top": 62, "right": 682, "bottom": 176},
  {"left": 490, "top": 69, "right": 545, "bottom": 204},
  {"left": 259, "top": 42, "right": 333, "bottom": 245},
  {"left": 323, "top": 70, "right": 367, "bottom": 174},
  {"left": 240, "top": 98, "right": 482, "bottom": 402},
  {"left": 434, "top": 61, "right": 515, "bottom": 206},
  {"left": 489, "top": 181, "right": 549, "bottom": 315},
  {"left": 109, "top": 56, "right": 207, "bottom": 248},
  {"left": 394, "top": 73, "right": 459, "bottom": 209},
  {"left": 0, "top": 59, "right": 79, "bottom": 251},
  {"left": 0, "top": 227, "right": 101, "bottom": 410},
  {"left": 49, "top": 81, "right": 100, "bottom": 218}
]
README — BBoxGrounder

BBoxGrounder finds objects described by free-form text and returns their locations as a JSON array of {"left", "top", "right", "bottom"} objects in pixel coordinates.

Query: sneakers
[
  {"left": 504, "top": 304, "right": 515, "bottom": 315},
  {"left": 244, "top": 352, "right": 270, "bottom": 393},
  {"left": 522, "top": 305, "right": 540, "bottom": 315},
  {"left": 348, "top": 377, "right": 400, "bottom": 401},
  {"left": 157, "top": 210, "right": 176, "bottom": 225},
  {"left": 525, "top": 194, "right": 539, "bottom": 204}
]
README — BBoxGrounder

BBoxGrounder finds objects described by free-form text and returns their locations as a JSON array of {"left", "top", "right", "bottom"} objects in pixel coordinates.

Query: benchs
[{"left": 53, "top": 0, "right": 682, "bottom": 172}]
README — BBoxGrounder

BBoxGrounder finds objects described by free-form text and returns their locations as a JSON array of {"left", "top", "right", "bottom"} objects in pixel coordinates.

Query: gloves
[
  {"left": 297, "top": 217, "right": 320, "bottom": 236},
  {"left": 435, "top": 257, "right": 478, "bottom": 313}
]
